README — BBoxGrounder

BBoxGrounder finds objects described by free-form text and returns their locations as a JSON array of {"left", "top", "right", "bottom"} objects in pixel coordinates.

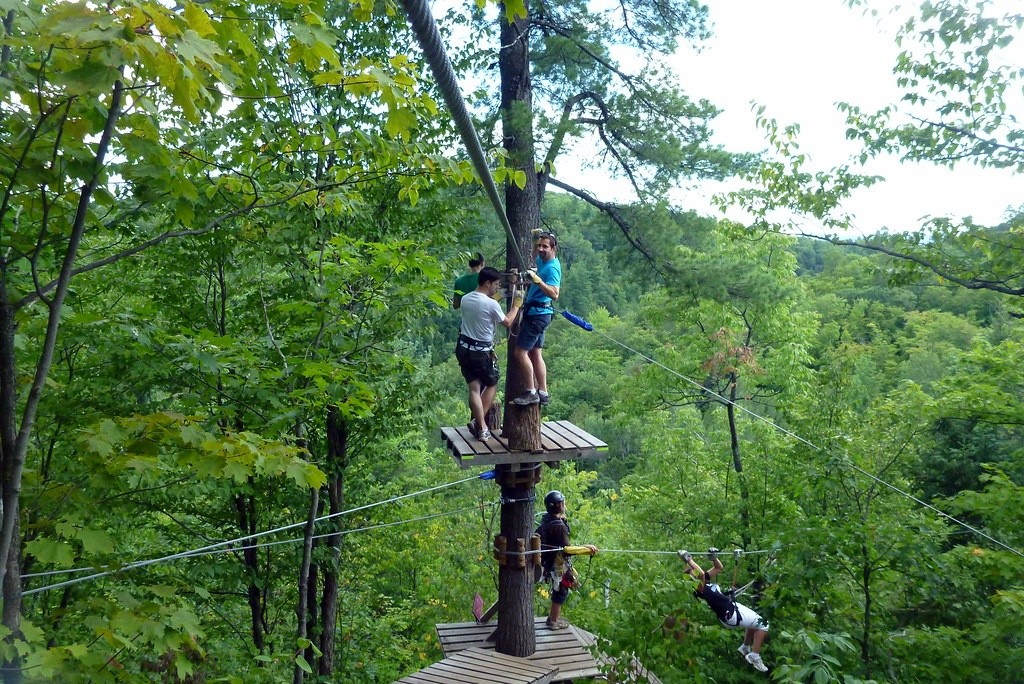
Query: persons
[
  {"left": 455, "top": 266, "right": 524, "bottom": 441},
  {"left": 452, "top": 252, "right": 485, "bottom": 311},
  {"left": 514, "top": 228, "right": 561, "bottom": 406},
  {"left": 675, "top": 547, "right": 769, "bottom": 672},
  {"left": 532, "top": 490, "right": 597, "bottom": 631}
]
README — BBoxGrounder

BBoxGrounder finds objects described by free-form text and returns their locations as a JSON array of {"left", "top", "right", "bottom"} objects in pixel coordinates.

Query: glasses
[{"left": 541, "top": 232, "right": 556, "bottom": 246}]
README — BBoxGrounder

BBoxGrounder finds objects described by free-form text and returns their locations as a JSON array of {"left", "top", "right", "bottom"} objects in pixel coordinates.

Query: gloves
[
  {"left": 524, "top": 270, "right": 542, "bottom": 283},
  {"left": 708, "top": 546, "right": 719, "bottom": 561},
  {"left": 678, "top": 549, "right": 692, "bottom": 563},
  {"left": 514, "top": 290, "right": 525, "bottom": 307},
  {"left": 492, "top": 288, "right": 509, "bottom": 302}
]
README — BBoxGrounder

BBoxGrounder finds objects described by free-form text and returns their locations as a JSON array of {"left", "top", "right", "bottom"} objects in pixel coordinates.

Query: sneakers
[
  {"left": 737, "top": 643, "right": 751, "bottom": 656},
  {"left": 550, "top": 619, "right": 569, "bottom": 630},
  {"left": 547, "top": 616, "right": 553, "bottom": 624},
  {"left": 537, "top": 389, "right": 550, "bottom": 404},
  {"left": 479, "top": 428, "right": 492, "bottom": 442},
  {"left": 467, "top": 418, "right": 478, "bottom": 438},
  {"left": 745, "top": 653, "right": 768, "bottom": 672},
  {"left": 514, "top": 391, "right": 540, "bottom": 405}
]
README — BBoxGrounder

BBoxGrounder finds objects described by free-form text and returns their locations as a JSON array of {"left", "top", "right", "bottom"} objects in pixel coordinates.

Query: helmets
[{"left": 545, "top": 490, "right": 566, "bottom": 514}]
